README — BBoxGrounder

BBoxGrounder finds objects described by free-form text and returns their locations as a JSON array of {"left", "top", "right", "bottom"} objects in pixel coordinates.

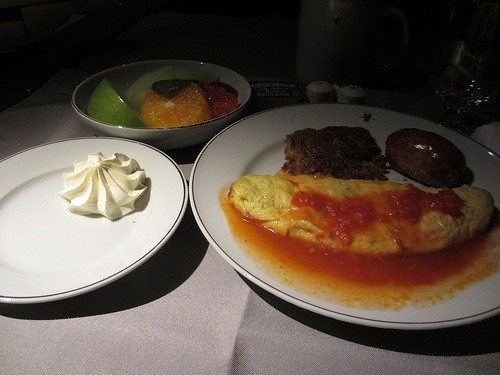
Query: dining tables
[{"left": 1, "top": 13, "right": 500, "bottom": 375}]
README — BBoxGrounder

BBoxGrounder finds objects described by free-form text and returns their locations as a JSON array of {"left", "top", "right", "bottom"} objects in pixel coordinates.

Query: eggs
[{"left": 227, "top": 171, "right": 495, "bottom": 256}]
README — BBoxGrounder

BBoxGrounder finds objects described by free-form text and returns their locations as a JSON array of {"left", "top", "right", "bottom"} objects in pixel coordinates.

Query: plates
[
  {"left": 0, "top": 136, "right": 188, "bottom": 304},
  {"left": 186, "top": 103, "right": 499, "bottom": 330},
  {"left": 70, "top": 58, "right": 251, "bottom": 149}
]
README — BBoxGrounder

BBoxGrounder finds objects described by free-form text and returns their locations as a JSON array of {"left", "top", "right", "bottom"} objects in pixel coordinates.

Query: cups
[{"left": 430, "top": 54, "right": 493, "bottom": 129}]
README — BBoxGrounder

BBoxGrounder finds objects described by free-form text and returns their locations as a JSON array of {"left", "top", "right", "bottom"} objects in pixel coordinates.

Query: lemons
[{"left": 88, "top": 77, "right": 145, "bottom": 128}]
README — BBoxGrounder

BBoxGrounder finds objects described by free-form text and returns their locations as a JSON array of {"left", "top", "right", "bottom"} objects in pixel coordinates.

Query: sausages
[{"left": 387, "top": 128, "right": 465, "bottom": 187}]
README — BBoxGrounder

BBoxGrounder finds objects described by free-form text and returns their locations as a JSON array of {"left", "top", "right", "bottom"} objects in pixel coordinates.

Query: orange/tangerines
[{"left": 141, "top": 84, "right": 213, "bottom": 128}]
